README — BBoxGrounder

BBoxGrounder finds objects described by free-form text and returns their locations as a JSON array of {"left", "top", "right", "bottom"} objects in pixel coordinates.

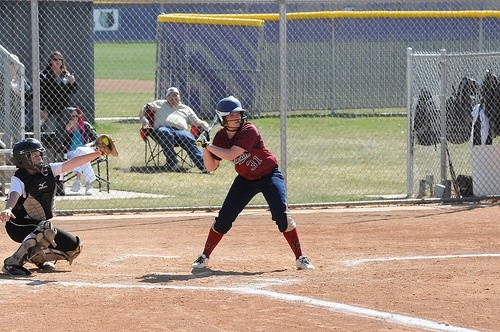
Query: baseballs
[{"left": 101, "top": 136, "right": 109, "bottom": 146}]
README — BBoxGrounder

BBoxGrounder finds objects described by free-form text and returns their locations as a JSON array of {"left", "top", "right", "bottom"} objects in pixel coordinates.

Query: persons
[
  {"left": 0, "top": 51, "right": 79, "bottom": 145},
  {"left": 192, "top": 97, "right": 315, "bottom": 270},
  {"left": 0, "top": 138, "right": 107, "bottom": 277},
  {"left": 139, "top": 87, "right": 219, "bottom": 173},
  {"left": 55, "top": 107, "right": 97, "bottom": 195}
]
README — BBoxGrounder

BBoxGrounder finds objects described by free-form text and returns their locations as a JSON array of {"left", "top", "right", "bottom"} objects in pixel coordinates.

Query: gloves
[{"left": 195, "top": 130, "right": 211, "bottom": 148}]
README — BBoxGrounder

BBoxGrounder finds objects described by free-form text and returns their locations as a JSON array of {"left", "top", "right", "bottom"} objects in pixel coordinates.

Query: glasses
[{"left": 52, "top": 58, "right": 63, "bottom": 61}]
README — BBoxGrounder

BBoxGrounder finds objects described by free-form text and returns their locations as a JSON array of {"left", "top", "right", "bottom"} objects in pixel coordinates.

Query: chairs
[
  {"left": 144, "top": 104, "right": 193, "bottom": 170},
  {"left": 54, "top": 139, "right": 111, "bottom": 195}
]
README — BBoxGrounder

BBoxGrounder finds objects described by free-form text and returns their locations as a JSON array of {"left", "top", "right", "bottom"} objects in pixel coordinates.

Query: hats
[{"left": 166, "top": 87, "right": 179, "bottom": 96}]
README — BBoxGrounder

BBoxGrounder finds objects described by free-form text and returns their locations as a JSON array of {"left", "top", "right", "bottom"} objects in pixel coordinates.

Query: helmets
[
  {"left": 216, "top": 97, "right": 245, "bottom": 126},
  {"left": 13, "top": 138, "right": 46, "bottom": 173}
]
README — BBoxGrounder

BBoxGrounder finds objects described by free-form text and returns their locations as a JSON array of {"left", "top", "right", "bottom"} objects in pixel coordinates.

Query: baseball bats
[
  {"left": 195, "top": 95, "right": 236, "bottom": 148},
  {"left": 442, "top": 139, "right": 461, "bottom": 198}
]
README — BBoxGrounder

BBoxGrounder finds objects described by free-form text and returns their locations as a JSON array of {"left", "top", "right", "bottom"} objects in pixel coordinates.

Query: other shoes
[
  {"left": 167, "top": 163, "right": 186, "bottom": 173},
  {"left": 202, "top": 163, "right": 219, "bottom": 173}
]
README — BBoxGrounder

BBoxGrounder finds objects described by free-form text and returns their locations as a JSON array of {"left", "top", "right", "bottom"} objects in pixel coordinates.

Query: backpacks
[
  {"left": 445, "top": 77, "right": 476, "bottom": 145},
  {"left": 413, "top": 87, "right": 441, "bottom": 145},
  {"left": 479, "top": 70, "right": 500, "bottom": 138}
]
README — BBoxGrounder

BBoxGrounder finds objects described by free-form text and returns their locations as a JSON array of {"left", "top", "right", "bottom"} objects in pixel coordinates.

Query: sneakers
[
  {"left": 28, "top": 259, "right": 56, "bottom": 270},
  {"left": 2, "top": 264, "right": 32, "bottom": 277},
  {"left": 70, "top": 181, "right": 80, "bottom": 192},
  {"left": 192, "top": 254, "right": 209, "bottom": 269},
  {"left": 296, "top": 256, "right": 315, "bottom": 270},
  {"left": 85, "top": 185, "right": 92, "bottom": 195}
]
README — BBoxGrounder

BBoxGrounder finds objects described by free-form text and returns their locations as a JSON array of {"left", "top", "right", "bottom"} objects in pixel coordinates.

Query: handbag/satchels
[
  {"left": 473, "top": 99, "right": 492, "bottom": 145},
  {"left": 453, "top": 175, "right": 473, "bottom": 197}
]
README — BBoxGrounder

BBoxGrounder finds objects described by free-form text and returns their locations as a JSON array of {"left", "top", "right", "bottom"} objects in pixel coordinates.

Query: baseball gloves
[{"left": 93, "top": 134, "right": 119, "bottom": 158}]
